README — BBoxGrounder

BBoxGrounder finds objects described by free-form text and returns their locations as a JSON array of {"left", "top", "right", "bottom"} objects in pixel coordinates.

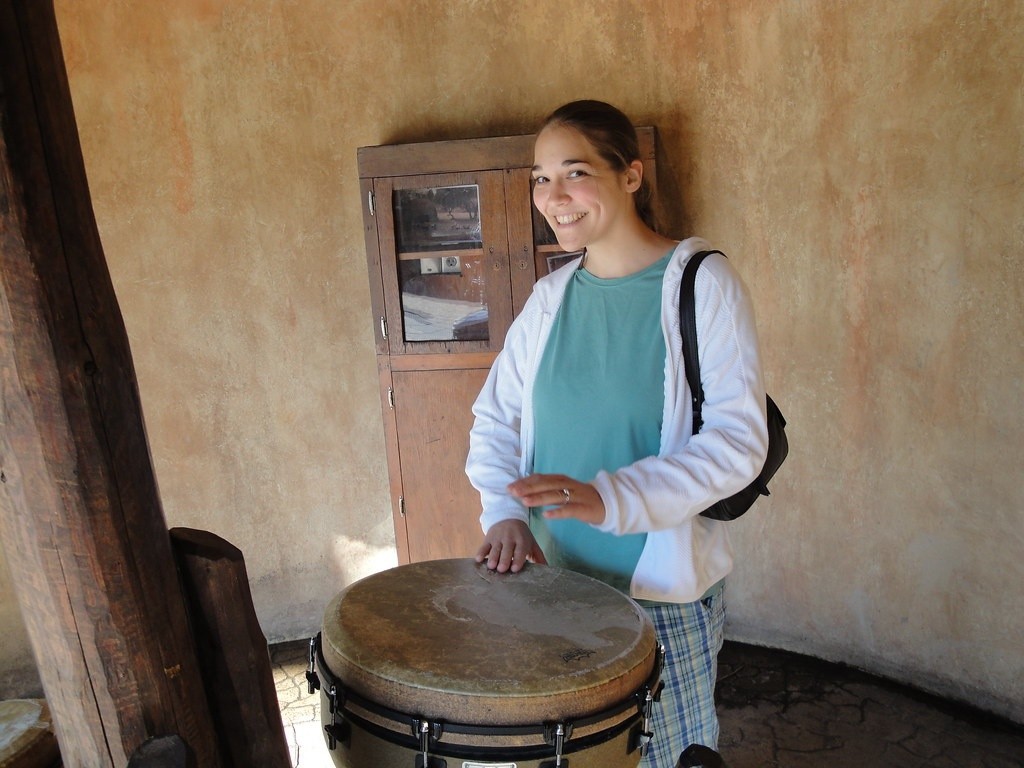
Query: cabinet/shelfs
[{"left": 356, "top": 122, "right": 661, "bottom": 568}]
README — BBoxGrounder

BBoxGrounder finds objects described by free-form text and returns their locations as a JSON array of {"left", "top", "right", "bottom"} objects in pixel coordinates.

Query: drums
[{"left": 307, "top": 558, "right": 667, "bottom": 768}]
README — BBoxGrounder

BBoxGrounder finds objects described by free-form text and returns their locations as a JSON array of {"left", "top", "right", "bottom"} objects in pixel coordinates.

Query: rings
[{"left": 563, "top": 489, "right": 569, "bottom": 505}]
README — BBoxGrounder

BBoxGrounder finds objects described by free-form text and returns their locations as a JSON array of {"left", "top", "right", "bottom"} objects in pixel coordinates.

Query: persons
[{"left": 464, "top": 101, "right": 769, "bottom": 768}]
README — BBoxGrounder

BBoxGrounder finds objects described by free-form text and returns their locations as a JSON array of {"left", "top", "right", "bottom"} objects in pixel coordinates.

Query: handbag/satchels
[{"left": 678, "top": 247, "right": 788, "bottom": 523}]
